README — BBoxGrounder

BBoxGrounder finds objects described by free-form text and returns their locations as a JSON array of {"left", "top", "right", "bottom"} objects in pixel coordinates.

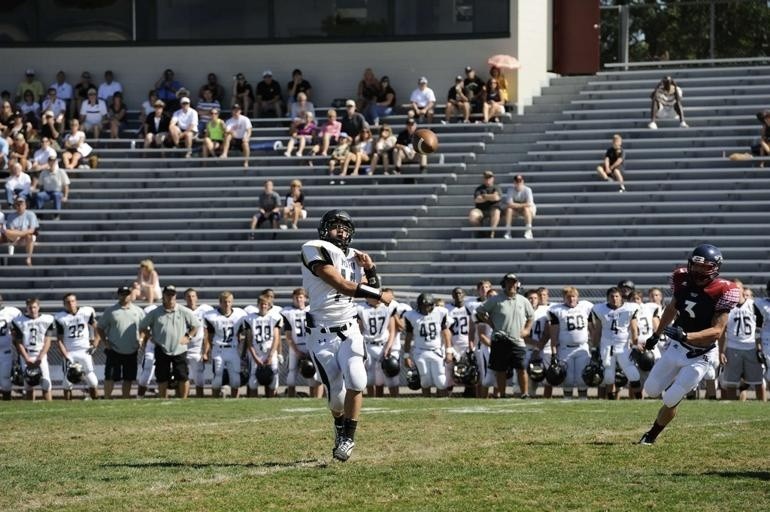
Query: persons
[
  {"left": 647, "top": 76, "right": 687, "bottom": 129},
  {"left": 0, "top": 257, "right": 328, "bottom": 400},
  {"left": 0, "top": 64, "right": 125, "bottom": 267},
  {"left": 359, "top": 272, "right": 675, "bottom": 400},
  {"left": 249, "top": 179, "right": 306, "bottom": 230},
  {"left": 469, "top": 169, "right": 536, "bottom": 239},
  {"left": 687, "top": 278, "right": 770, "bottom": 401},
  {"left": 140, "top": 67, "right": 508, "bottom": 185},
  {"left": 299, "top": 208, "right": 394, "bottom": 459},
  {"left": 596, "top": 135, "right": 628, "bottom": 193},
  {"left": 758, "top": 110, "right": 770, "bottom": 167},
  {"left": 634, "top": 242, "right": 741, "bottom": 446}
]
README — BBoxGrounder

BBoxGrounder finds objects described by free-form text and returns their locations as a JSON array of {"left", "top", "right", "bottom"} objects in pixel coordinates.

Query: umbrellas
[{"left": 487, "top": 54, "right": 520, "bottom": 76}]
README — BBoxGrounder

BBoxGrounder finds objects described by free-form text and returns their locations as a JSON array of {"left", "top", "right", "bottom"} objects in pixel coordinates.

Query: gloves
[
  {"left": 645, "top": 332, "right": 660, "bottom": 350},
  {"left": 664, "top": 325, "right": 687, "bottom": 342}
]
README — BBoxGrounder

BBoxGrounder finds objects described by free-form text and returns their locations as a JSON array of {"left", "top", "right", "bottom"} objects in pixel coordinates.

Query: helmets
[
  {"left": 301, "top": 360, "right": 318, "bottom": 378},
  {"left": 629, "top": 348, "right": 656, "bottom": 369},
  {"left": 580, "top": 363, "right": 606, "bottom": 387},
  {"left": 417, "top": 293, "right": 434, "bottom": 309},
  {"left": 381, "top": 357, "right": 421, "bottom": 390},
  {"left": 617, "top": 280, "right": 635, "bottom": 299},
  {"left": 22, "top": 364, "right": 43, "bottom": 386},
  {"left": 528, "top": 359, "right": 567, "bottom": 385},
  {"left": 254, "top": 364, "right": 273, "bottom": 385},
  {"left": 317, "top": 208, "right": 355, "bottom": 245},
  {"left": 687, "top": 244, "right": 722, "bottom": 283},
  {"left": 450, "top": 359, "right": 481, "bottom": 382},
  {"left": 66, "top": 364, "right": 83, "bottom": 383}
]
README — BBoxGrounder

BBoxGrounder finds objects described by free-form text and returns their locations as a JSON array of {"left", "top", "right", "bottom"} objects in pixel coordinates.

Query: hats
[
  {"left": 14, "top": 67, "right": 97, "bottom": 140},
  {"left": 262, "top": 69, "right": 273, "bottom": 77},
  {"left": 482, "top": 168, "right": 524, "bottom": 182},
  {"left": 417, "top": 66, "right": 474, "bottom": 82},
  {"left": 346, "top": 99, "right": 356, "bottom": 106},
  {"left": 154, "top": 87, "right": 241, "bottom": 110},
  {"left": 116, "top": 285, "right": 132, "bottom": 294},
  {"left": 164, "top": 283, "right": 177, "bottom": 294},
  {"left": 503, "top": 273, "right": 518, "bottom": 287}
]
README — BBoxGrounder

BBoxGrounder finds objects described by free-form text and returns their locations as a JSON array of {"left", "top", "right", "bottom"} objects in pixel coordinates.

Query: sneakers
[
  {"left": 648, "top": 120, "right": 690, "bottom": 128},
  {"left": 279, "top": 223, "right": 298, "bottom": 230},
  {"left": 504, "top": 229, "right": 534, "bottom": 239},
  {"left": 286, "top": 149, "right": 430, "bottom": 177},
  {"left": 640, "top": 430, "right": 654, "bottom": 446},
  {"left": 330, "top": 423, "right": 354, "bottom": 461}
]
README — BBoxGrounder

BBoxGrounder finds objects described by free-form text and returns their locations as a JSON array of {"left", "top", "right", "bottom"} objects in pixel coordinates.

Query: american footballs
[{"left": 412, "top": 129, "right": 437, "bottom": 154}]
